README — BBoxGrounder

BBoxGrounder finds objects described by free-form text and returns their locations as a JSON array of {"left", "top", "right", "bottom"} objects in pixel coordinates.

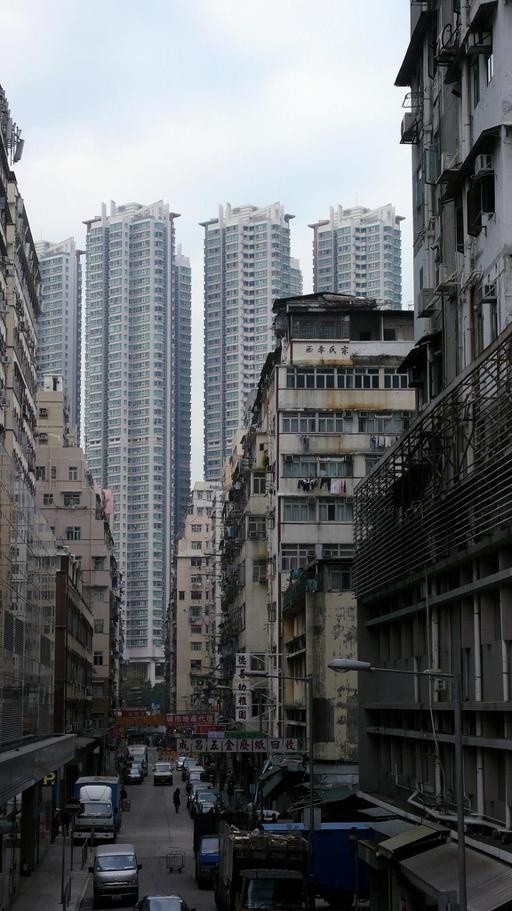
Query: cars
[
  {"left": 175, "top": 755, "right": 221, "bottom": 890},
  {"left": 124, "top": 744, "right": 173, "bottom": 786}
]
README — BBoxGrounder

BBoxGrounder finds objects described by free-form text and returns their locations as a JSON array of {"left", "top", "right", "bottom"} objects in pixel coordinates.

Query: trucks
[
  {"left": 215, "top": 826, "right": 311, "bottom": 911},
  {"left": 71, "top": 774, "right": 121, "bottom": 842}
]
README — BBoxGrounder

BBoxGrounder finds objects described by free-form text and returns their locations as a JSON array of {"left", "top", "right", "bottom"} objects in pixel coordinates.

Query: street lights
[
  {"left": 326, "top": 657, "right": 469, "bottom": 911},
  {"left": 244, "top": 670, "right": 316, "bottom": 845}
]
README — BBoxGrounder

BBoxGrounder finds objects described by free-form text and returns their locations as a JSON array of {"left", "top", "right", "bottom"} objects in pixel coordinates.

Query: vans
[{"left": 92, "top": 843, "right": 139, "bottom": 908}]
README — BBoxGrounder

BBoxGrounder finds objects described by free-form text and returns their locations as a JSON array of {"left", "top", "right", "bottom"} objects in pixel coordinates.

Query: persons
[
  {"left": 258, "top": 814, "right": 268, "bottom": 828},
  {"left": 270, "top": 814, "right": 277, "bottom": 824},
  {"left": 172, "top": 788, "right": 181, "bottom": 814},
  {"left": 53, "top": 807, "right": 62, "bottom": 836},
  {"left": 120, "top": 786, "right": 128, "bottom": 809},
  {"left": 204, "top": 760, "right": 236, "bottom": 807},
  {"left": 60, "top": 806, "right": 72, "bottom": 837}
]
